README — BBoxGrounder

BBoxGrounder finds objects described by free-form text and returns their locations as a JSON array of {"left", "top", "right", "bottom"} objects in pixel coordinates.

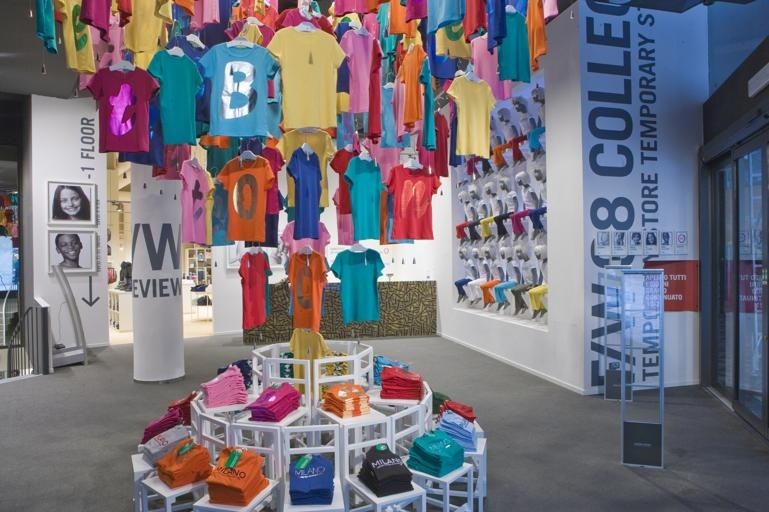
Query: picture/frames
[
  {"left": 48, "top": 181, "right": 98, "bottom": 227},
  {"left": 48, "top": 229, "right": 98, "bottom": 274}
]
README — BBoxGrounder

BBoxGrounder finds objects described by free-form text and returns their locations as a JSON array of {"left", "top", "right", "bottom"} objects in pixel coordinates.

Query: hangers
[{"left": 105, "top": 2, "right": 518, "bottom": 260}]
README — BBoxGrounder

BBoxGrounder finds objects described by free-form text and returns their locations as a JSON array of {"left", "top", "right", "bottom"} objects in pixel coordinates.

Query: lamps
[
  {"left": 27, "top": 0, "right": 33, "bottom": 19},
  {"left": 41, "top": 43, "right": 49, "bottom": 77},
  {"left": 72, "top": 71, "right": 81, "bottom": 97}
]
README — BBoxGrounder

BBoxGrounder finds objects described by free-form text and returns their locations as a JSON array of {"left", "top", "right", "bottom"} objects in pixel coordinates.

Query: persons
[
  {"left": 55, "top": 234, "right": 85, "bottom": 267},
  {"left": 452, "top": 85, "right": 549, "bottom": 320},
  {"left": 52, "top": 184, "right": 91, "bottom": 222}
]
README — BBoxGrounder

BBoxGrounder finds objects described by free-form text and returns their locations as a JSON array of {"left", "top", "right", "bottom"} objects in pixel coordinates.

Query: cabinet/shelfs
[
  {"left": 184, "top": 241, "right": 213, "bottom": 286},
  {"left": 107, "top": 288, "right": 134, "bottom": 333},
  {"left": 127, "top": 341, "right": 488, "bottom": 512}
]
